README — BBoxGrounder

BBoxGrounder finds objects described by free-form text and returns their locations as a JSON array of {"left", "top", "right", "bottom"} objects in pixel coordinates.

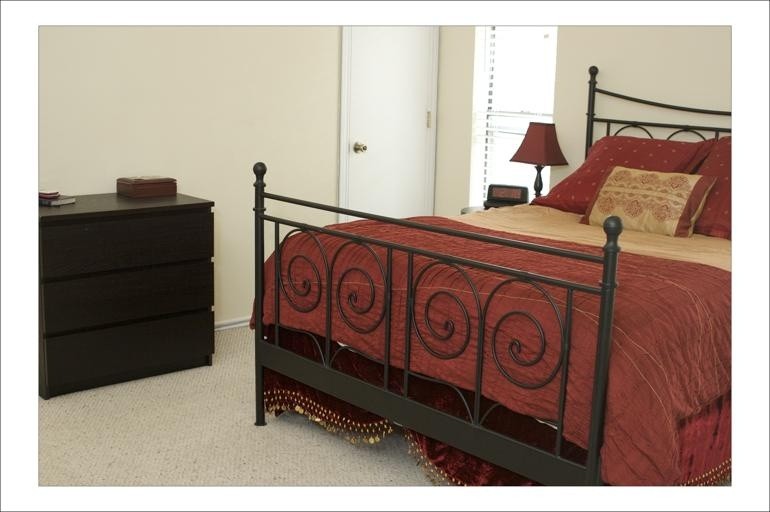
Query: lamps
[{"left": 510, "top": 122, "right": 569, "bottom": 198}]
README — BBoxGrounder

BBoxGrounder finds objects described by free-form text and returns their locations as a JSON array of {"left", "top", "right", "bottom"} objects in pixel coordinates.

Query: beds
[{"left": 249, "top": 66, "right": 732, "bottom": 486}]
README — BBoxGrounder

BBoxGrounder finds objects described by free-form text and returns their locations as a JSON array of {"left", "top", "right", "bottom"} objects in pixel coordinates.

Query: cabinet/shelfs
[{"left": 39, "top": 191, "right": 215, "bottom": 400}]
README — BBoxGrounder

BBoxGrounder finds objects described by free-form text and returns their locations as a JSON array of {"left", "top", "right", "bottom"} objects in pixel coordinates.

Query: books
[
  {"left": 116, "top": 177, "right": 177, "bottom": 199},
  {"left": 39, "top": 192, "right": 76, "bottom": 207}
]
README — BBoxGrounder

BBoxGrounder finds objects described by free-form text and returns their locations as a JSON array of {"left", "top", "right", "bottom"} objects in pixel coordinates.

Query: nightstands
[{"left": 460, "top": 206, "right": 484, "bottom": 214}]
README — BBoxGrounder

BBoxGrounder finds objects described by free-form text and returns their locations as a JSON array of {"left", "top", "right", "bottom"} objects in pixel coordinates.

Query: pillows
[
  {"left": 578, "top": 165, "right": 718, "bottom": 237},
  {"left": 693, "top": 136, "right": 731, "bottom": 239},
  {"left": 529, "top": 135, "right": 713, "bottom": 214}
]
